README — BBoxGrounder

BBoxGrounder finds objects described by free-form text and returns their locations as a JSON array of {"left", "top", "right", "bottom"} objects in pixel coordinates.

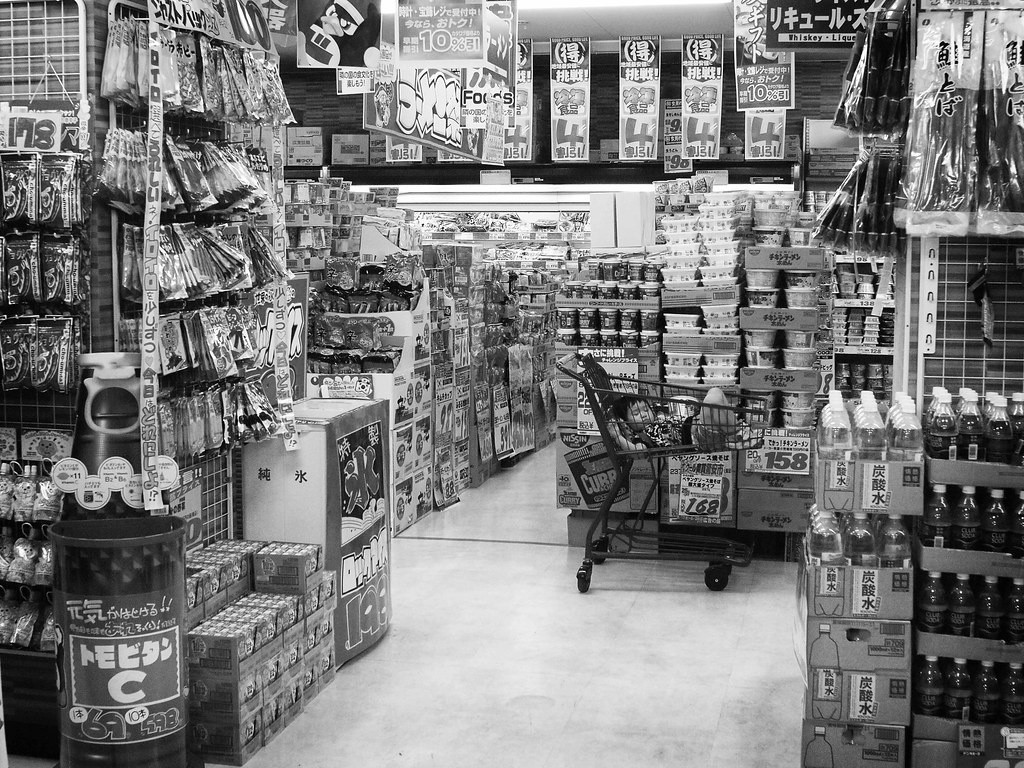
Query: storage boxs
[
  {"left": 553, "top": 237, "right": 1023, "bottom": 767},
  {"left": 186, "top": 538, "right": 334, "bottom": 765},
  {"left": 231, "top": 118, "right": 803, "bottom": 165},
  {"left": 289, "top": 204, "right": 433, "bottom": 540}
]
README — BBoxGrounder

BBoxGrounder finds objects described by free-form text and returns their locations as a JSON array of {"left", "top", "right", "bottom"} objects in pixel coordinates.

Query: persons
[{"left": 608, "top": 387, "right": 766, "bottom": 453}]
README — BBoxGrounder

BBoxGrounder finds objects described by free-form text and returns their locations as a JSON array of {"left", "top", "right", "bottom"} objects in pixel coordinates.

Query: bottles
[
  {"left": 818, "top": 387, "right": 1024, "bottom": 467},
  {"left": 629, "top": 458, "right": 655, "bottom": 510},
  {"left": 846, "top": 628, "right": 872, "bottom": 642},
  {"left": 806, "top": 503, "right": 912, "bottom": 570},
  {"left": 833, "top": 724, "right": 866, "bottom": 746},
  {"left": 917, "top": 571, "right": 1024, "bottom": 645},
  {"left": 917, "top": 655, "right": 1024, "bottom": 729},
  {"left": 919, "top": 484, "right": 1024, "bottom": 559}
]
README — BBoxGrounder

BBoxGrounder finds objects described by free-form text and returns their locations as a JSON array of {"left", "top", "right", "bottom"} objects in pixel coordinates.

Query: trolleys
[{"left": 556, "top": 352, "right": 770, "bottom": 592}]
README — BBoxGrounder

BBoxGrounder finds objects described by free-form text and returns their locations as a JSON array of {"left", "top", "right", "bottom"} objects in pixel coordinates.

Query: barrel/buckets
[{"left": 49, "top": 516, "right": 186, "bottom": 768}]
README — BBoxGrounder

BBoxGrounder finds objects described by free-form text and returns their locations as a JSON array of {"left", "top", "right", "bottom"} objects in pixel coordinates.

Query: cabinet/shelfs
[{"left": 826, "top": 240, "right": 896, "bottom": 412}]
